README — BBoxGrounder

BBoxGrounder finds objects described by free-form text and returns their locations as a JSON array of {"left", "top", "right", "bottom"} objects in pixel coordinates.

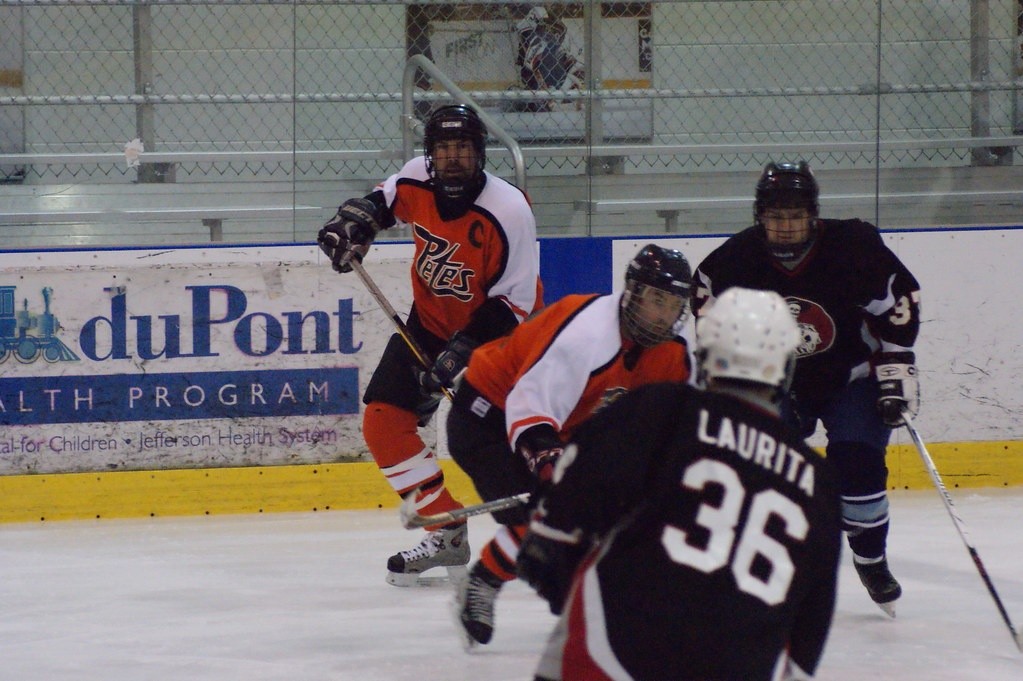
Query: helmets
[
  {"left": 620, "top": 244, "right": 697, "bottom": 348},
  {"left": 693, "top": 286, "right": 802, "bottom": 386},
  {"left": 423, "top": 104, "right": 487, "bottom": 201},
  {"left": 752, "top": 161, "right": 819, "bottom": 262}
]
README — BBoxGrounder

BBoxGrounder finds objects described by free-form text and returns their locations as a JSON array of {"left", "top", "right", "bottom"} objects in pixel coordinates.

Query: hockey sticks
[
  {"left": 901, "top": 412, "right": 1023, "bottom": 653},
  {"left": 397, "top": 489, "right": 533, "bottom": 531},
  {"left": 349, "top": 255, "right": 454, "bottom": 403}
]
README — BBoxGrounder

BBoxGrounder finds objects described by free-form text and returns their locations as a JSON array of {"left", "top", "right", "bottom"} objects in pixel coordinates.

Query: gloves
[
  {"left": 317, "top": 198, "right": 386, "bottom": 273},
  {"left": 515, "top": 519, "right": 590, "bottom": 616},
  {"left": 514, "top": 423, "right": 568, "bottom": 498},
  {"left": 411, "top": 330, "right": 478, "bottom": 392},
  {"left": 870, "top": 351, "right": 920, "bottom": 428}
]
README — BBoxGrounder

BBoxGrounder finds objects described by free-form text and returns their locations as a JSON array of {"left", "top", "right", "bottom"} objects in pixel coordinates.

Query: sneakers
[
  {"left": 459, "top": 559, "right": 506, "bottom": 647},
  {"left": 385, "top": 521, "right": 471, "bottom": 588},
  {"left": 853, "top": 552, "right": 903, "bottom": 618}
]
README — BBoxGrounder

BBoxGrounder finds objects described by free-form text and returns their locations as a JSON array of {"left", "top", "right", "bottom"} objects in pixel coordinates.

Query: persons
[
  {"left": 514, "top": 285, "right": 842, "bottom": 681},
  {"left": 448, "top": 245, "right": 694, "bottom": 654},
  {"left": 688, "top": 162, "right": 921, "bottom": 618},
  {"left": 317, "top": 103, "right": 547, "bottom": 586},
  {"left": 503, "top": 3, "right": 584, "bottom": 112}
]
